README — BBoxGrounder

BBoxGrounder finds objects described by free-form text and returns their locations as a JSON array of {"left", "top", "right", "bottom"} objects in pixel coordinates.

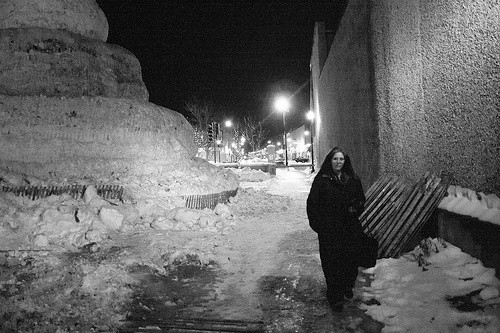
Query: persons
[{"left": 307, "top": 147, "right": 365, "bottom": 312}]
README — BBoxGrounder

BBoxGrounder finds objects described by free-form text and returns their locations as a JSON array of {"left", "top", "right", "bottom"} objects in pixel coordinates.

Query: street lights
[
  {"left": 307, "top": 110, "right": 316, "bottom": 173},
  {"left": 274, "top": 93, "right": 290, "bottom": 167},
  {"left": 225, "top": 120, "right": 232, "bottom": 162}
]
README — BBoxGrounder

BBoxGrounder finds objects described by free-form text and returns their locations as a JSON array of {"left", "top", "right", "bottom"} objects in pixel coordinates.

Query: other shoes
[
  {"left": 333, "top": 304, "right": 344, "bottom": 312},
  {"left": 344, "top": 286, "right": 353, "bottom": 299}
]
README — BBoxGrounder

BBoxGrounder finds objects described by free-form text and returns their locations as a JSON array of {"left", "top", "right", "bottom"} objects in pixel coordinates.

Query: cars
[{"left": 295, "top": 156, "right": 308, "bottom": 163}]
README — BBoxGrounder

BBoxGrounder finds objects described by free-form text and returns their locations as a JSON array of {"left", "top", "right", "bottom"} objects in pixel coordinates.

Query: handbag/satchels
[{"left": 356, "top": 234, "right": 379, "bottom": 268}]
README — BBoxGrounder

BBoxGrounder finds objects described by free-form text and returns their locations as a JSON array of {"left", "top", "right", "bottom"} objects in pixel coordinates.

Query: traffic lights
[{"left": 206, "top": 122, "right": 220, "bottom": 145}]
